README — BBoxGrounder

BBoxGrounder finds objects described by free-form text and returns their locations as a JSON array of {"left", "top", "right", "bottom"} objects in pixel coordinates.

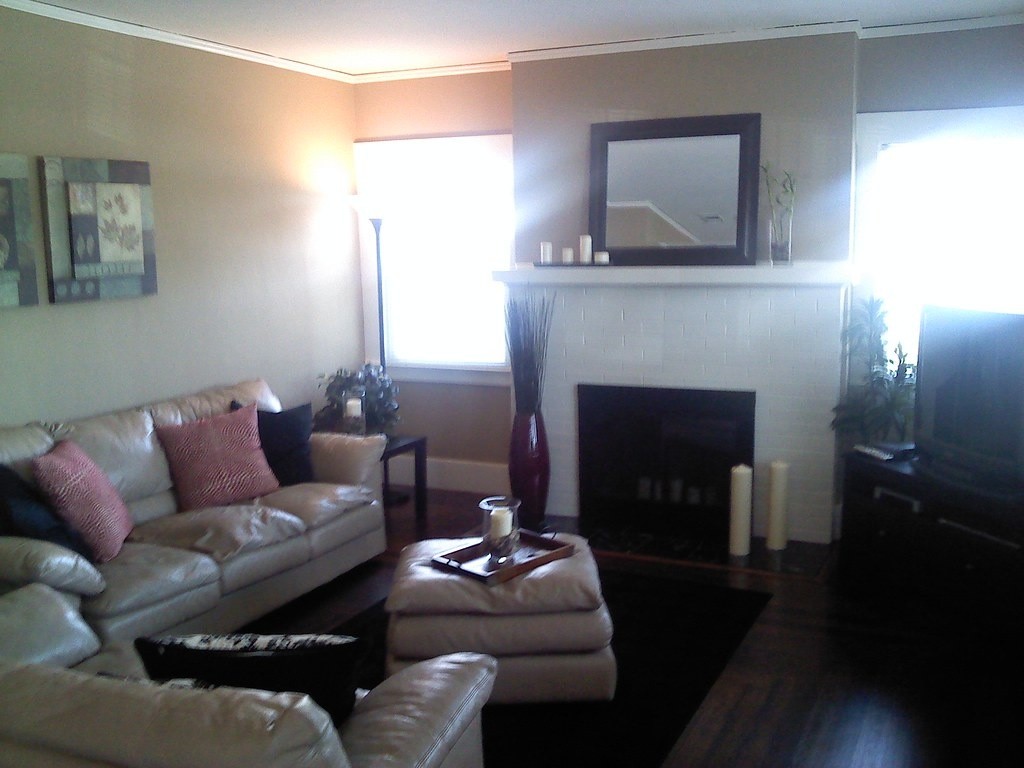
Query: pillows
[
  {"left": 33, "top": 437, "right": 135, "bottom": 562},
  {"left": 227, "top": 398, "right": 316, "bottom": 483},
  {"left": 98, "top": 631, "right": 359, "bottom": 734},
  {"left": 160, "top": 408, "right": 278, "bottom": 511},
  {"left": 0, "top": 463, "right": 90, "bottom": 557}
]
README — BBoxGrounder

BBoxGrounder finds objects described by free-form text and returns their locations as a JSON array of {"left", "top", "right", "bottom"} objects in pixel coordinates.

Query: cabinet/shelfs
[{"left": 842, "top": 445, "right": 1024, "bottom": 607}]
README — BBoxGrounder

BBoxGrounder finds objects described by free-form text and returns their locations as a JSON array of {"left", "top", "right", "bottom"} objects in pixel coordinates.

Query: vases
[
  {"left": 766, "top": 194, "right": 794, "bottom": 266},
  {"left": 510, "top": 407, "right": 550, "bottom": 524}
]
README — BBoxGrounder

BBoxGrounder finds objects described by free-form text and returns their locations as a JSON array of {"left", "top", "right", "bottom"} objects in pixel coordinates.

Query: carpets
[{"left": 236, "top": 572, "right": 776, "bottom": 767}]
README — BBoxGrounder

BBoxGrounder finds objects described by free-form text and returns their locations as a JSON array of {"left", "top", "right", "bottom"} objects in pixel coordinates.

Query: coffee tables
[{"left": 363, "top": 435, "right": 429, "bottom": 548}]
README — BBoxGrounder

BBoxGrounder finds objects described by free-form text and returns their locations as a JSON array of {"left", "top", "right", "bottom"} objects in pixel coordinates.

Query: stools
[{"left": 384, "top": 534, "right": 617, "bottom": 703}]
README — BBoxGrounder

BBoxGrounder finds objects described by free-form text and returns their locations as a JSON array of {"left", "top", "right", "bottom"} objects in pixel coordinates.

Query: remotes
[{"left": 854, "top": 444, "right": 894, "bottom": 462}]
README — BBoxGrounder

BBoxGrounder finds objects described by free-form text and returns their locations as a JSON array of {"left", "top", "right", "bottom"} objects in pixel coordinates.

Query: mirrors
[{"left": 587, "top": 111, "right": 761, "bottom": 267}]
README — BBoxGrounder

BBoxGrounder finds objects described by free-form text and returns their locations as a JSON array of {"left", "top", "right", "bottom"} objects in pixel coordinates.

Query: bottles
[{"left": 479, "top": 495, "right": 521, "bottom": 567}]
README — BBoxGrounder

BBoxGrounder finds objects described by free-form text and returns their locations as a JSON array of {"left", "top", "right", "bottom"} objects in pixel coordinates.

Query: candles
[
  {"left": 347, "top": 397, "right": 363, "bottom": 418},
  {"left": 489, "top": 505, "right": 513, "bottom": 542},
  {"left": 594, "top": 251, "right": 609, "bottom": 266},
  {"left": 765, "top": 458, "right": 787, "bottom": 550},
  {"left": 562, "top": 247, "right": 573, "bottom": 267},
  {"left": 579, "top": 234, "right": 592, "bottom": 264},
  {"left": 540, "top": 241, "right": 552, "bottom": 264},
  {"left": 729, "top": 463, "right": 754, "bottom": 556}
]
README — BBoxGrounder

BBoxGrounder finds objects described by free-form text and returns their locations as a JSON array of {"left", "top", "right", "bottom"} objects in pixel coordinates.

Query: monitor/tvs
[{"left": 910, "top": 306, "right": 1024, "bottom": 509}]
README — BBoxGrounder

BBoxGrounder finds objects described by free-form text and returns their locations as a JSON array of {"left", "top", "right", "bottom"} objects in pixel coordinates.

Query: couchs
[
  {"left": 0, "top": 649, "right": 508, "bottom": 768},
  {"left": 0, "top": 376, "right": 388, "bottom": 678}
]
